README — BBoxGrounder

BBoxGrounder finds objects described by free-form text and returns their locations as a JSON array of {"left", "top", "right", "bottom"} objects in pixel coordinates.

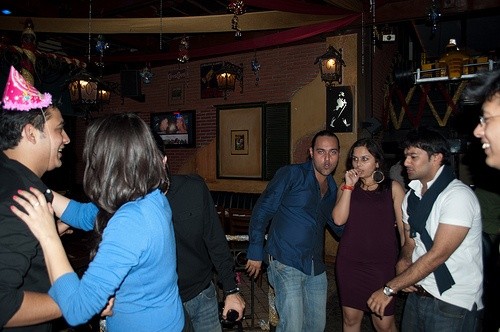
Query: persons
[
  {"left": 151, "top": 126, "right": 245, "bottom": 332},
  {"left": 0, "top": 98, "right": 114, "bottom": 332},
  {"left": 247, "top": 129, "right": 342, "bottom": 332},
  {"left": 11, "top": 112, "right": 191, "bottom": 332},
  {"left": 364, "top": 128, "right": 485, "bottom": 332},
  {"left": 176, "top": 117, "right": 188, "bottom": 133},
  {"left": 473, "top": 76, "right": 500, "bottom": 170},
  {"left": 331, "top": 139, "right": 408, "bottom": 332}
]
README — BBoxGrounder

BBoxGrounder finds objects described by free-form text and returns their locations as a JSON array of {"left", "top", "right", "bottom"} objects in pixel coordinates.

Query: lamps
[
  {"left": 97, "top": 85, "right": 112, "bottom": 113},
  {"left": 212, "top": 61, "right": 244, "bottom": 101},
  {"left": 313, "top": 44, "right": 347, "bottom": 92},
  {"left": 67, "top": 79, "right": 100, "bottom": 126}
]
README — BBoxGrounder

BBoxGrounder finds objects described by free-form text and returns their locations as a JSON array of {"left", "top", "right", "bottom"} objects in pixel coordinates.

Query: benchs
[{"left": 208, "top": 190, "right": 263, "bottom": 235}]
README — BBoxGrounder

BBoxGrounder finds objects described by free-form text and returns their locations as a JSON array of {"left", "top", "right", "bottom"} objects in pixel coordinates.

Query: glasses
[{"left": 479, "top": 115, "right": 499, "bottom": 126}]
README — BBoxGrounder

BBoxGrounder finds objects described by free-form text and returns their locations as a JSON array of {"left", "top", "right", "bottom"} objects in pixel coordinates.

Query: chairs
[
  {"left": 225, "top": 206, "right": 262, "bottom": 288},
  {"left": 213, "top": 204, "right": 224, "bottom": 235}
]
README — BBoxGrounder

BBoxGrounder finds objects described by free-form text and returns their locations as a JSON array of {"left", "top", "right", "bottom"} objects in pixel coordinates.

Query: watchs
[{"left": 383, "top": 283, "right": 398, "bottom": 296}]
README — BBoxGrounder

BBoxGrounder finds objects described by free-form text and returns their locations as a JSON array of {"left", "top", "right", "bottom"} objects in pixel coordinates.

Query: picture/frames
[{"left": 213, "top": 100, "right": 267, "bottom": 182}]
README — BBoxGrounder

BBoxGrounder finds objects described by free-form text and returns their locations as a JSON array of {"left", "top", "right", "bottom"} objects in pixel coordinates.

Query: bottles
[
  {"left": 218, "top": 302, "right": 239, "bottom": 328},
  {"left": 469, "top": 53, "right": 477, "bottom": 74},
  {"left": 424, "top": 59, "right": 448, "bottom": 77},
  {"left": 488, "top": 50, "right": 497, "bottom": 70}
]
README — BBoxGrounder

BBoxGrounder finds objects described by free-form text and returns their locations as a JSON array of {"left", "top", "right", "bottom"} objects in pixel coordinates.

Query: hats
[{"left": 3, "top": 66, "right": 52, "bottom": 109}]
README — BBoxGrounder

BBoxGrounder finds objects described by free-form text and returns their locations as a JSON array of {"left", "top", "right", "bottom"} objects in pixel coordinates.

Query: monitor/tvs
[{"left": 149, "top": 109, "right": 197, "bottom": 148}]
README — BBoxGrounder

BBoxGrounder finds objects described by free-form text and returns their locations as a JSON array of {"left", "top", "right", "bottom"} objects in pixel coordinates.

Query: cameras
[{"left": 218, "top": 301, "right": 240, "bottom": 328}]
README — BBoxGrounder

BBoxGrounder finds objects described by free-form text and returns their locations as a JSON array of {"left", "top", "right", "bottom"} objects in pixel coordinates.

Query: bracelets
[
  {"left": 225, "top": 287, "right": 240, "bottom": 296},
  {"left": 341, "top": 185, "right": 355, "bottom": 190}
]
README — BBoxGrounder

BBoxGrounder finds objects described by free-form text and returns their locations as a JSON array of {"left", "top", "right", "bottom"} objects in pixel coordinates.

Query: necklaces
[{"left": 362, "top": 182, "right": 377, "bottom": 190}]
